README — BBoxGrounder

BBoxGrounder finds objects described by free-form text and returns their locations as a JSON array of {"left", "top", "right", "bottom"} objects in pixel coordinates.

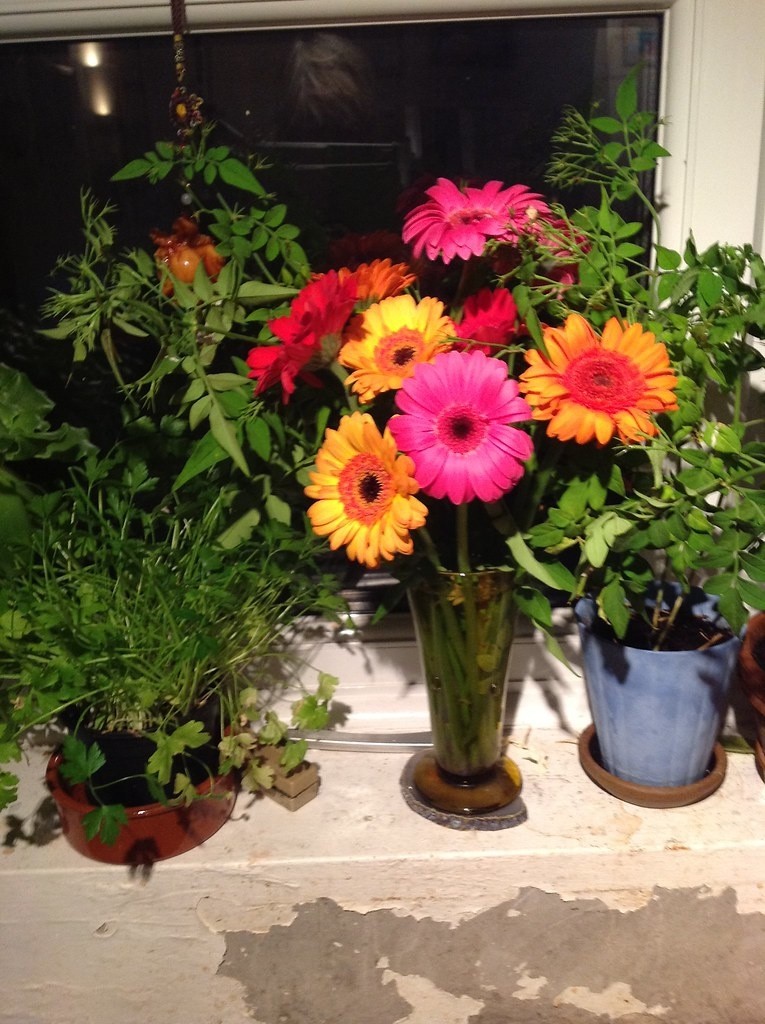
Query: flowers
[{"left": 250, "top": 177, "right": 681, "bottom": 566}]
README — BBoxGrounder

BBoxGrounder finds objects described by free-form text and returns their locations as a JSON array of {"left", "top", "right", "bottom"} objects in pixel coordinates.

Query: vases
[{"left": 405, "top": 563, "right": 527, "bottom": 830}]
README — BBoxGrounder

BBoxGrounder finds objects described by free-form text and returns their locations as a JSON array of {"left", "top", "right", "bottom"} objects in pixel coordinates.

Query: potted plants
[
  {"left": 2, "top": 121, "right": 354, "bottom": 868},
  {"left": 494, "top": 64, "right": 764, "bottom": 809}
]
